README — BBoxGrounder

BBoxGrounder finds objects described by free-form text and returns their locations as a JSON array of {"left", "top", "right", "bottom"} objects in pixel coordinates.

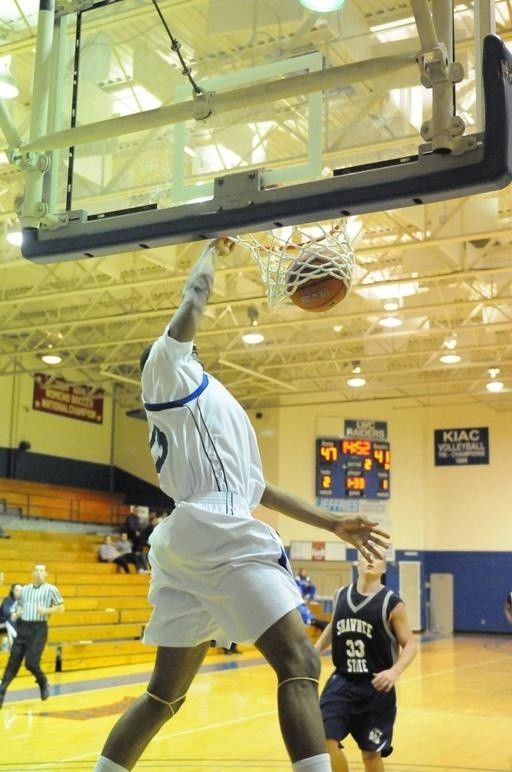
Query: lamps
[
  {"left": 439, "top": 335, "right": 461, "bottom": 364},
  {"left": 241, "top": 304, "right": 265, "bottom": 345},
  {"left": 347, "top": 360, "right": 366, "bottom": 387},
  {"left": 379, "top": 298, "right": 403, "bottom": 327},
  {"left": 486, "top": 367, "right": 504, "bottom": 392},
  {"left": 41, "top": 334, "right": 61, "bottom": 365}
]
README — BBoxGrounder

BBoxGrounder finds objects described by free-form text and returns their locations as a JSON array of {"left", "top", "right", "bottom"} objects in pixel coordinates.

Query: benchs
[
  {"left": 1, "top": 478, "right": 129, "bottom": 525},
  {"left": 0, "top": 528, "right": 333, "bottom": 690}
]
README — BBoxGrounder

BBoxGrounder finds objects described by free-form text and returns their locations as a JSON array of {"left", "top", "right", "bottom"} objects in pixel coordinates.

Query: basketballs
[{"left": 286, "top": 251, "right": 347, "bottom": 312}]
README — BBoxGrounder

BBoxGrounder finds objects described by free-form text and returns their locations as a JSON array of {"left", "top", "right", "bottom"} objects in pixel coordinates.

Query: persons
[
  {"left": 98, "top": 500, "right": 171, "bottom": 577},
  {"left": 0, "top": 565, "right": 65, "bottom": 710},
  {"left": 92, "top": 236, "right": 391, "bottom": 772},
  {"left": 290, "top": 540, "right": 419, "bottom": 772}
]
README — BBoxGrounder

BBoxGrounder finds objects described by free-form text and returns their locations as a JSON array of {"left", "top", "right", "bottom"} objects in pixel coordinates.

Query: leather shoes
[{"left": 40, "top": 682, "right": 50, "bottom": 701}]
